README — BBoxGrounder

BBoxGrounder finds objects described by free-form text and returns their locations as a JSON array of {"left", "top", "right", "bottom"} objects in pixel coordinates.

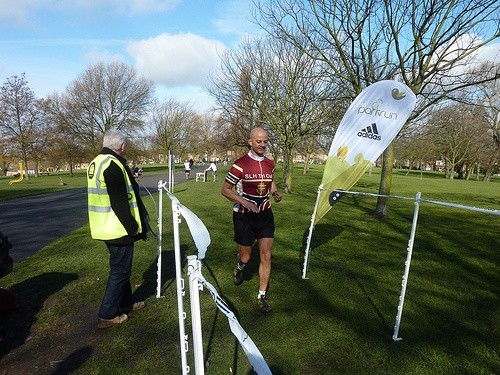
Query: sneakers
[
  {"left": 233, "top": 261, "right": 246, "bottom": 285},
  {"left": 257, "top": 295, "right": 272, "bottom": 314}
]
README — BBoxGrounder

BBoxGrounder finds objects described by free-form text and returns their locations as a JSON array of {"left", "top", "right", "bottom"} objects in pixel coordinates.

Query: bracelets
[{"left": 272, "top": 191, "right": 277, "bottom": 197}]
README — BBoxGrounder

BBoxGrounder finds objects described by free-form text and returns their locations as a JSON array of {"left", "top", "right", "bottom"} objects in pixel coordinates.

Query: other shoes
[
  {"left": 119, "top": 299, "right": 145, "bottom": 313},
  {"left": 97, "top": 314, "right": 127, "bottom": 329}
]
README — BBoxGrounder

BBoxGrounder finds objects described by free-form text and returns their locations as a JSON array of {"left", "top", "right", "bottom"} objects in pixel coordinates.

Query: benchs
[{"left": 196, "top": 170, "right": 207, "bottom": 182}]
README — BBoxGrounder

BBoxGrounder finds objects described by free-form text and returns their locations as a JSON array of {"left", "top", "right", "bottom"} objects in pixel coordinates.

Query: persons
[
  {"left": 87, "top": 129, "right": 158, "bottom": 329},
  {"left": 221, "top": 127, "right": 283, "bottom": 314},
  {"left": 183, "top": 154, "right": 228, "bottom": 180}
]
